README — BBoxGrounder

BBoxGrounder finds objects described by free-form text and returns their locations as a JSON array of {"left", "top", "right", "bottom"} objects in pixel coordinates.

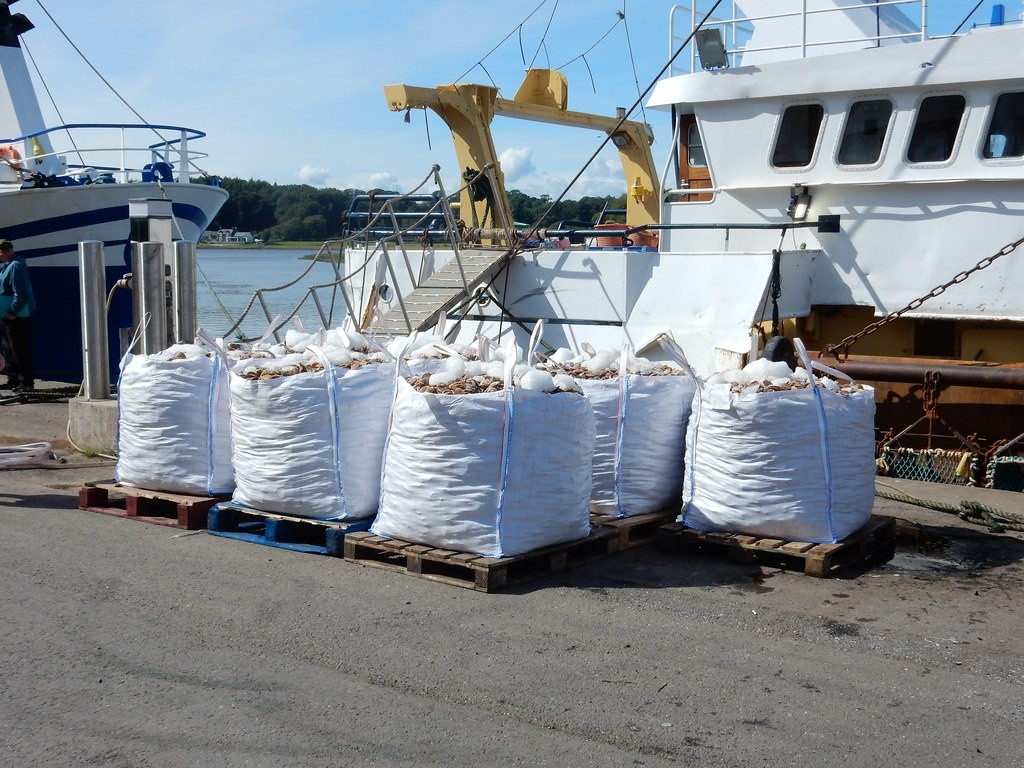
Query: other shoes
[
  {"left": 0, "top": 379, "right": 19, "bottom": 390},
  {"left": 13, "top": 383, "right": 34, "bottom": 392}
]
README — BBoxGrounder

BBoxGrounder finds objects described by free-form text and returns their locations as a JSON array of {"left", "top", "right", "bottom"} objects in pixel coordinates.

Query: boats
[
  {"left": 338, "top": 0, "right": 1023, "bottom": 487},
  {"left": 1, "top": 0, "right": 230, "bottom": 381}
]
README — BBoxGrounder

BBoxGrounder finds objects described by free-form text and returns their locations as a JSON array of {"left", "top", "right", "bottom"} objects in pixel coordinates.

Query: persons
[{"left": 0, "top": 239, "right": 35, "bottom": 393}]
